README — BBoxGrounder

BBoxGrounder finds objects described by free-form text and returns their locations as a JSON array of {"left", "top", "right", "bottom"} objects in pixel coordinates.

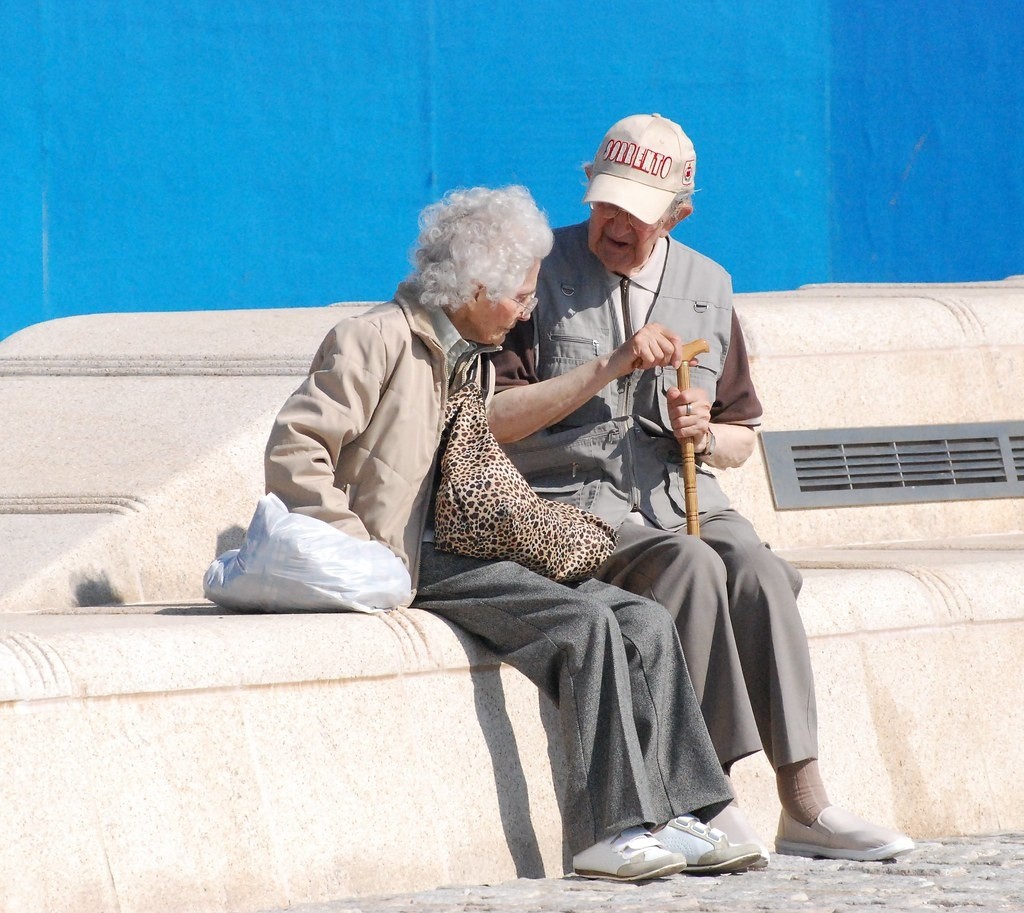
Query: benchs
[{"left": 1, "top": 534, "right": 1024, "bottom": 913}]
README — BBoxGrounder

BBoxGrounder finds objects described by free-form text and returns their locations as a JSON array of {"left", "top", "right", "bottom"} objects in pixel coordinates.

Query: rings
[{"left": 686, "top": 404, "right": 691, "bottom": 415}]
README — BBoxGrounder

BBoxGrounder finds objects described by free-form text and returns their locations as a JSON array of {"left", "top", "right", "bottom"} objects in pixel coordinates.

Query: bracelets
[{"left": 695, "top": 427, "right": 715, "bottom": 460}]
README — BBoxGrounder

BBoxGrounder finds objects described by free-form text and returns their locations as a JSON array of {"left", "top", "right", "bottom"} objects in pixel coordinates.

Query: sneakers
[
  {"left": 646, "top": 813, "right": 761, "bottom": 875},
  {"left": 573, "top": 814, "right": 687, "bottom": 880},
  {"left": 693, "top": 796, "right": 770, "bottom": 869},
  {"left": 774, "top": 805, "right": 916, "bottom": 860}
]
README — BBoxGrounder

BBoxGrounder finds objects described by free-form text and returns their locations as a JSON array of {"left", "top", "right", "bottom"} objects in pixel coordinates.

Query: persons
[
  {"left": 265, "top": 183, "right": 759, "bottom": 884},
  {"left": 484, "top": 112, "right": 916, "bottom": 866}
]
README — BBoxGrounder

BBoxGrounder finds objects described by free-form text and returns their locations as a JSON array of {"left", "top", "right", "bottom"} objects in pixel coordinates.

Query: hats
[{"left": 583, "top": 114, "right": 696, "bottom": 225}]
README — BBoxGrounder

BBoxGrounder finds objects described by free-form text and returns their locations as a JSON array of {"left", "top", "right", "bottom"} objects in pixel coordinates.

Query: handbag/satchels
[
  {"left": 201, "top": 495, "right": 412, "bottom": 615},
  {"left": 437, "top": 382, "right": 621, "bottom": 584}
]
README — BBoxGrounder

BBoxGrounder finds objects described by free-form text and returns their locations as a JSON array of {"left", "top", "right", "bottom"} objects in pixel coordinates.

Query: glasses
[
  {"left": 502, "top": 292, "right": 539, "bottom": 318},
  {"left": 587, "top": 198, "right": 680, "bottom": 232}
]
